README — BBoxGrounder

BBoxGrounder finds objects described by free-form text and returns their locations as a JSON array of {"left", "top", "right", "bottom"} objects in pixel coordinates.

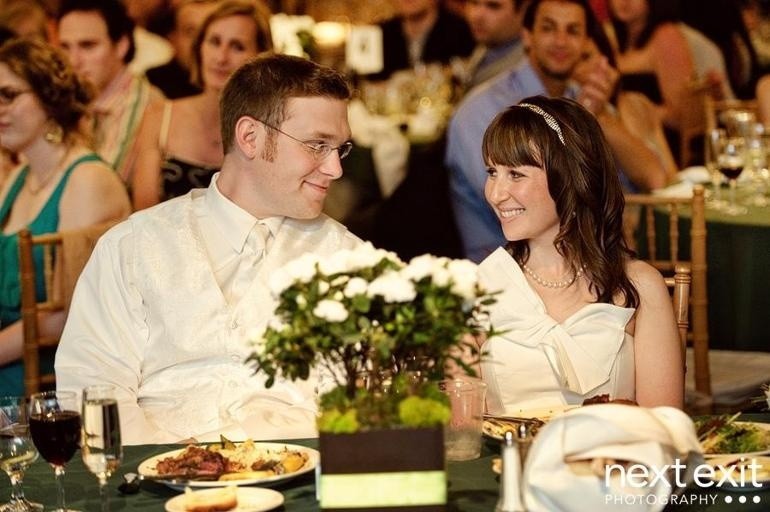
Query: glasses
[
  {"left": 0, "top": 86, "right": 34, "bottom": 105},
  {"left": 255, "top": 118, "right": 353, "bottom": 163}
]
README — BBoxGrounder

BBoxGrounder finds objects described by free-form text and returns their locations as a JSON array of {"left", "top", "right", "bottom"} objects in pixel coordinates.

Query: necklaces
[{"left": 517, "top": 258, "right": 588, "bottom": 290}]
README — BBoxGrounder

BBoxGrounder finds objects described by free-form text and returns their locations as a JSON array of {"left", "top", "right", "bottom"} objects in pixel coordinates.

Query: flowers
[{"left": 240, "top": 238, "right": 514, "bottom": 428}]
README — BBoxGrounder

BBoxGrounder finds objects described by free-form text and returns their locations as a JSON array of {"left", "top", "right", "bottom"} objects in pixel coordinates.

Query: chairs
[
  {"left": 621, "top": 183, "right": 770, "bottom": 409},
  {"left": 681, "top": 84, "right": 712, "bottom": 164},
  {"left": 14, "top": 229, "right": 72, "bottom": 400},
  {"left": 661, "top": 266, "right": 696, "bottom": 358}
]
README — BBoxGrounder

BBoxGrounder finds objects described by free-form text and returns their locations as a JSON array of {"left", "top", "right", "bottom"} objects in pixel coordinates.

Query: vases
[{"left": 312, "top": 421, "right": 451, "bottom": 511}]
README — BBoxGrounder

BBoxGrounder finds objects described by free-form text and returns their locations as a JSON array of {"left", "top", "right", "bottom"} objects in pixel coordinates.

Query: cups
[{"left": 443, "top": 380, "right": 487, "bottom": 461}]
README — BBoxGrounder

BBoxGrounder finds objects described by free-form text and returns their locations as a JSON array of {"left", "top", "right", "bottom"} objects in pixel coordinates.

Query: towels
[{"left": 523, "top": 403, "right": 705, "bottom": 512}]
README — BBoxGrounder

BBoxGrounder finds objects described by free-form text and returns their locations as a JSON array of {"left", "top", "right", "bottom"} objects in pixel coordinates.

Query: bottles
[{"left": 496, "top": 423, "right": 531, "bottom": 511}]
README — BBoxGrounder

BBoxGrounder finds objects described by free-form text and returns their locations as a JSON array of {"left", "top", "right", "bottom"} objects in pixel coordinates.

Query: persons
[
  {"left": 453, "top": 93, "right": 687, "bottom": 417},
  {"left": 2, "top": 36, "right": 131, "bottom": 411},
  {"left": 0, "top": 0, "right": 770, "bottom": 265},
  {"left": 53, "top": 52, "right": 408, "bottom": 447}
]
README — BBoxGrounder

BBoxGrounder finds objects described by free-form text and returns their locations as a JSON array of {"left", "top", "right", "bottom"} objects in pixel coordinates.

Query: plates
[
  {"left": 482, "top": 406, "right": 580, "bottom": 442},
  {"left": 164, "top": 487, "right": 285, "bottom": 512},
  {"left": 704, "top": 457, "right": 770, "bottom": 492},
  {"left": 137, "top": 442, "right": 320, "bottom": 486},
  {"left": 694, "top": 422, "right": 770, "bottom": 457}
]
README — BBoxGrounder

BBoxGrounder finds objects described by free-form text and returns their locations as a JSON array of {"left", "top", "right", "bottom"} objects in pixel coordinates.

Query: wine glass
[
  {"left": 78, "top": 383, "right": 122, "bottom": 512},
  {"left": 704, "top": 109, "right": 769, "bottom": 216},
  {"left": 0, "top": 397, "right": 43, "bottom": 512},
  {"left": 27, "top": 390, "right": 81, "bottom": 512}
]
